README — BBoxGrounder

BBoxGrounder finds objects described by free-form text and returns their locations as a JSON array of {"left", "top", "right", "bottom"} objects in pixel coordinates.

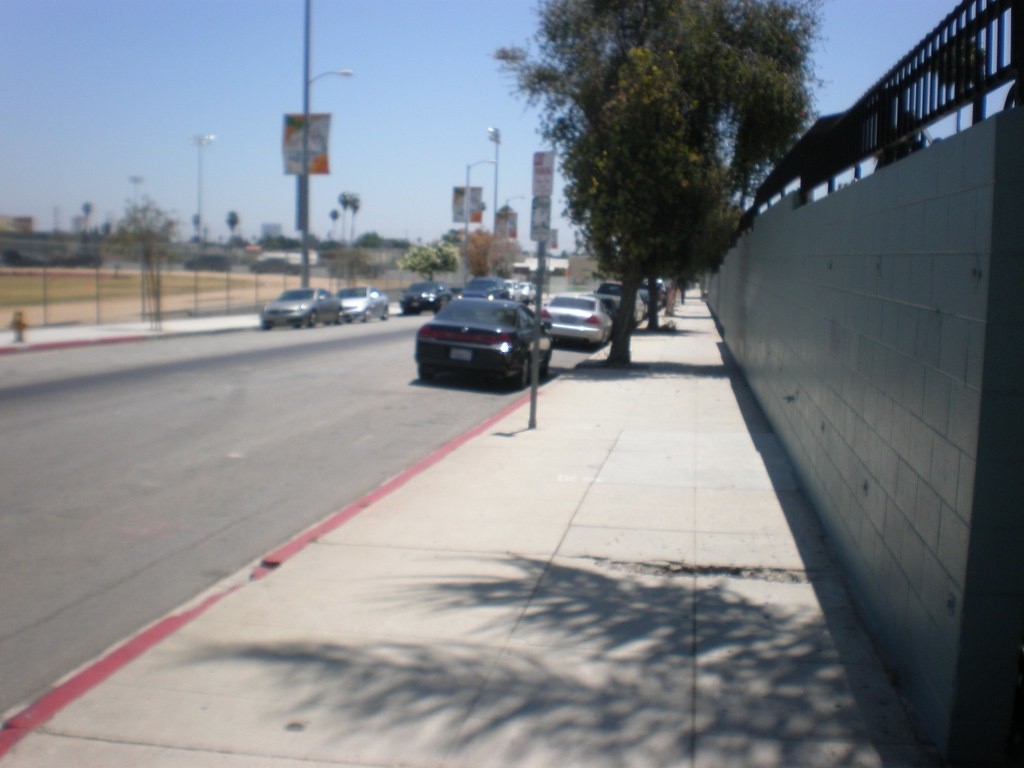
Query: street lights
[
  {"left": 463, "top": 159, "right": 499, "bottom": 294},
  {"left": 189, "top": 135, "right": 215, "bottom": 313},
  {"left": 485, "top": 125, "right": 500, "bottom": 276},
  {"left": 298, "top": 68, "right": 354, "bottom": 286}
]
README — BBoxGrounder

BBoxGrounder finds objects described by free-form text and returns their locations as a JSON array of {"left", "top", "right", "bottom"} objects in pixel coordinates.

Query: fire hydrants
[{"left": 7, "top": 310, "right": 27, "bottom": 343}]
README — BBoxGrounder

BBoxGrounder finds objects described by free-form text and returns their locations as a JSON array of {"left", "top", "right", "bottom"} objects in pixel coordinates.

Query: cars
[
  {"left": 588, "top": 278, "right": 671, "bottom": 329},
  {"left": 541, "top": 293, "right": 614, "bottom": 347},
  {"left": 414, "top": 298, "right": 555, "bottom": 391},
  {"left": 333, "top": 286, "right": 390, "bottom": 324},
  {"left": 258, "top": 286, "right": 342, "bottom": 329},
  {"left": 397, "top": 280, "right": 454, "bottom": 315},
  {"left": 461, "top": 275, "right": 537, "bottom": 307}
]
